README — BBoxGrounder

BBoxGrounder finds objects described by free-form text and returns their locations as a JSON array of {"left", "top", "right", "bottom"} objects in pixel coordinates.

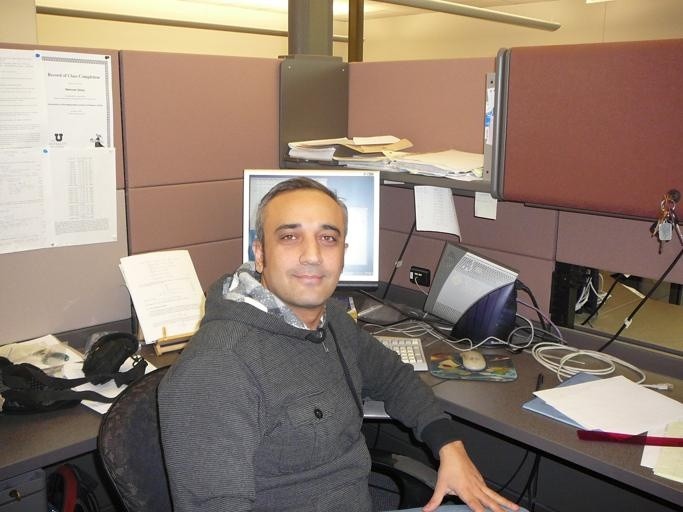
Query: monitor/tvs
[{"left": 243, "top": 168, "right": 379, "bottom": 289}]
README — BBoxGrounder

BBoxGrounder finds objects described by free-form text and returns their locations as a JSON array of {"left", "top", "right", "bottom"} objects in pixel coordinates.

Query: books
[
  {"left": 119, "top": 249, "right": 205, "bottom": 344},
  {"left": 532, "top": 375, "right": 682, "bottom": 440},
  {"left": 520, "top": 371, "right": 602, "bottom": 432},
  {"left": 287, "top": 135, "right": 484, "bottom": 182}
]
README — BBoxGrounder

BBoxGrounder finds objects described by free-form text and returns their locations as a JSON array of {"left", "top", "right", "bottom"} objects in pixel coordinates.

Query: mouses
[{"left": 461, "top": 350, "right": 486, "bottom": 372}]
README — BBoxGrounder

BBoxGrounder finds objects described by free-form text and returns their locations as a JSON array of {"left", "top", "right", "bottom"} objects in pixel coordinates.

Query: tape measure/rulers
[{"left": 577, "top": 430, "right": 683, "bottom": 447}]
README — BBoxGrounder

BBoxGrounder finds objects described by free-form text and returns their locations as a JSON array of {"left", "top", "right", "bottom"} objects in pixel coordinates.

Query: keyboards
[{"left": 378, "top": 335, "right": 428, "bottom": 371}]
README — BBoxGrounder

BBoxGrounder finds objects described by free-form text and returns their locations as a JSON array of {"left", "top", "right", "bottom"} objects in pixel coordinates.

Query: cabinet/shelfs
[
  {"left": 1, "top": 316, "right": 683, "bottom": 512},
  {"left": 276, "top": 36, "right": 683, "bottom": 223}
]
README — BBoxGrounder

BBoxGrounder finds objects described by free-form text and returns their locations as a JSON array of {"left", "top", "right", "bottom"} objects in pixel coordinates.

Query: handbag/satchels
[{"left": 0, "top": 356, "right": 81, "bottom": 415}]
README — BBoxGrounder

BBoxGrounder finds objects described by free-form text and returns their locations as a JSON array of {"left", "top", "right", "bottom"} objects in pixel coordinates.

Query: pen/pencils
[{"left": 535, "top": 374, "right": 543, "bottom": 397}]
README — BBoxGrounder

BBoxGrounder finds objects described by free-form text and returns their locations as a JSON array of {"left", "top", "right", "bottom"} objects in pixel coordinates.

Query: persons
[{"left": 156, "top": 176, "right": 532, "bottom": 512}]
